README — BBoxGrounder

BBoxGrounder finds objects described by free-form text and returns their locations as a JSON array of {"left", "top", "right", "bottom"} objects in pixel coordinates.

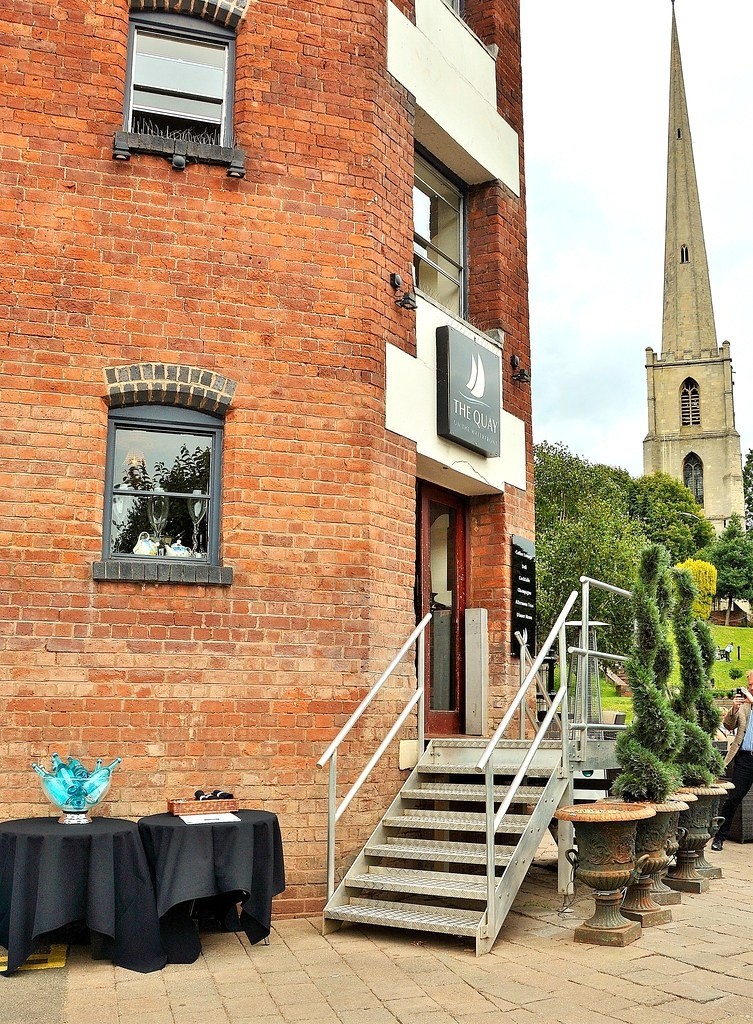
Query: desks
[
  {"left": 139, "top": 808, "right": 285, "bottom": 964},
  {"left": 0, "top": 817, "right": 168, "bottom": 977}
]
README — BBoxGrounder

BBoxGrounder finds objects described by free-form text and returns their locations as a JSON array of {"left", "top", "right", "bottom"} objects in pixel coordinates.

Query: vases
[{"left": 554, "top": 802, "right": 656, "bottom": 946}]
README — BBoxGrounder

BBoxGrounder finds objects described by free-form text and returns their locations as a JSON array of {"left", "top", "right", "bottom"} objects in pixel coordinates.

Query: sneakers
[{"left": 711, "top": 835, "right": 724, "bottom": 851}]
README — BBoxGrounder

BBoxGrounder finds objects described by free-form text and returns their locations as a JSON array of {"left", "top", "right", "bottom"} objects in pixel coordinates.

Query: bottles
[{"left": 31, "top": 752, "right": 122, "bottom": 810}]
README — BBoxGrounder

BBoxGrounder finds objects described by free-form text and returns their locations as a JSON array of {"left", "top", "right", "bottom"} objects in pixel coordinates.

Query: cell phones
[{"left": 737, "top": 689, "right": 744, "bottom": 699}]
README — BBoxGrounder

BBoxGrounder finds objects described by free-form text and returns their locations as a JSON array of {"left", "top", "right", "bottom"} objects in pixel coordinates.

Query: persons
[
  {"left": 712, "top": 671, "right": 753, "bottom": 850},
  {"left": 725, "top": 642, "right": 733, "bottom": 662}
]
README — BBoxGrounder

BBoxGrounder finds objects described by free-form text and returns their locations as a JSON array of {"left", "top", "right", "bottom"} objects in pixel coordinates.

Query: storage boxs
[{"left": 165, "top": 796, "right": 239, "bottom": 816}]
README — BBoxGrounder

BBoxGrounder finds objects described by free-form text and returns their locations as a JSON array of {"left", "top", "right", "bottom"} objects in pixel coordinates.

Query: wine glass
[
  {"left": 147, "top": 487, "right": 169, "bottom": 548},
  {"left": 187, "top": 490, "right": 208, "bottom": 558}
]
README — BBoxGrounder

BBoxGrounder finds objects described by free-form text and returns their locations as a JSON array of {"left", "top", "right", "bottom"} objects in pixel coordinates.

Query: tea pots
[
  {"left": 132, "top": 531, "right": 160, "bottom": 557},
  {"left": 164, "top": 540, "right": 191, "bottom": 558}
]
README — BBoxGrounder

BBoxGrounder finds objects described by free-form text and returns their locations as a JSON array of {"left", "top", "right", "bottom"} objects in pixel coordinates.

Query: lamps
[
  {"left": 510, "top": 355, "right": 530, "bottom": 383},
  {"left": 113, "top": 140, "right": 132, "bottom": 162},
  {"left": 601, "top": 711, "right": 626, "bottom": 739},
  {"left": 225, "top": 159, "right": 245, "bottom": 178},
  {"left": 390, "top": 272, "right": 418, "bottom": 310}
]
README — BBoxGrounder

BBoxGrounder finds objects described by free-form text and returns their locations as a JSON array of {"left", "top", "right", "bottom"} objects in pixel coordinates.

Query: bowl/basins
[{"left": 41, "top": 777, "right": 112, "bottom": 824}]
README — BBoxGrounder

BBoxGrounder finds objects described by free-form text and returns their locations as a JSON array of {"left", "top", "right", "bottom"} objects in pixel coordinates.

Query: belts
[{"left": 739, "top": 750, "right": 753, "bottom": 755}]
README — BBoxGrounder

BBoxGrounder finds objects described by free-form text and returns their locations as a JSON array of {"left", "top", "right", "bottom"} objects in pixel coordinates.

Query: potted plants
[{"left": 609, "top": 545, "right": 736, "bottom": 928}]
